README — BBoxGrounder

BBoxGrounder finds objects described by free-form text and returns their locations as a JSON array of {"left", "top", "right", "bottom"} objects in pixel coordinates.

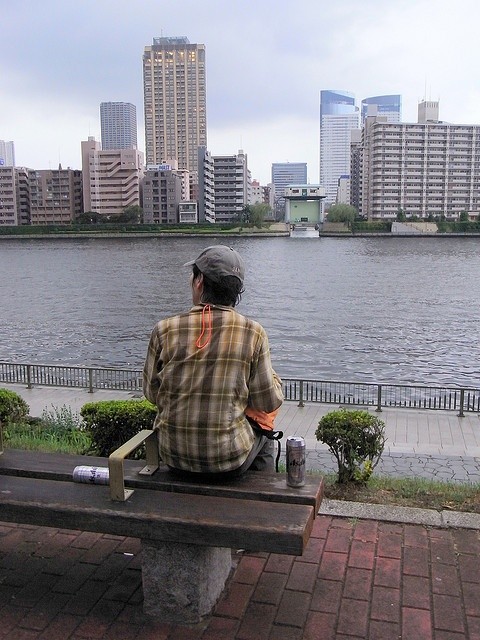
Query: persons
[{"left": 142, "top": 244, "right": 285, "bottom": 482}]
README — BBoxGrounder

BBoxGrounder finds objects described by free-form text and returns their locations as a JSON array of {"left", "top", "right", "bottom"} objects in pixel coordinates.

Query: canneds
[
  {"left": 73, "top": 466, "right": 110, "bottom": 485},
  {"left": 286, "top": 436, "right": 307, "bottom": 488}
]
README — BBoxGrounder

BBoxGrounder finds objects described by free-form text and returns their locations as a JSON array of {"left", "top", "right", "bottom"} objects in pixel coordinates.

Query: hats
[{"left": 183, "top": 245, "right": 245, "bottom": 288}]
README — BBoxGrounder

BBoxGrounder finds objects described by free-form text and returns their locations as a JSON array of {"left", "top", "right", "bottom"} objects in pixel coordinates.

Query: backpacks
[{"left": 244, "top": 406, "right": 283, "bottom": 473}]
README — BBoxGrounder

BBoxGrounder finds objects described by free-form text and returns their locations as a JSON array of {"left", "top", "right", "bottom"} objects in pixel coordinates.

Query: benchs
[{"left": 0, "top": 429, "right": 324, "bottom": 624}]
